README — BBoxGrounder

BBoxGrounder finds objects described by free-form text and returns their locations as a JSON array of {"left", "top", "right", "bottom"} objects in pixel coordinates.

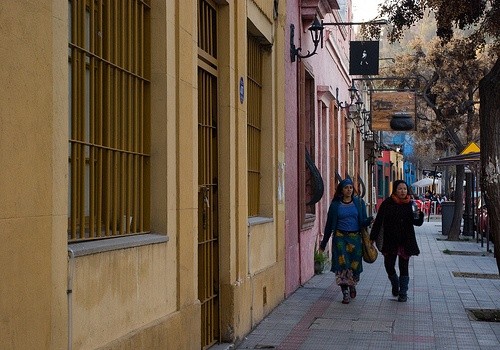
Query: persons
[
  {"left": 410, "top": 190, "right": 444, "bottom": 215},
  {"left": 317, "top": 179, "right": 375, "bottom": 304},
  {"left": 369, "top": 179, "right": 424, "bottom": 302}
]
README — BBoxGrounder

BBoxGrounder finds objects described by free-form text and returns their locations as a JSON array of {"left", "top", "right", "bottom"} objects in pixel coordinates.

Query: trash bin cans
[{"left": 441, "top": 201, "right": 455, "bottom": 235}]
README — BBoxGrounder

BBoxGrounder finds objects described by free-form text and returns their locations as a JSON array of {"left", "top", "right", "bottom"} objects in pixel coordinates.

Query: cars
[{"left": 461, "top": 166, "right": 495, "bottom": 243}]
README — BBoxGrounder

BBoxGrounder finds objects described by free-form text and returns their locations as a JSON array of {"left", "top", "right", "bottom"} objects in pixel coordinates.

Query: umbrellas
[{"left": 412, "top": 177, "right": 439, "bottom": 190}]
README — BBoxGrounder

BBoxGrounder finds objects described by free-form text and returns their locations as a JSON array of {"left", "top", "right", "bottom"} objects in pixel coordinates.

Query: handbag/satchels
[
  {"left": 314, "top": 247, "right": 330, "bottom": 273},
  {"left": 362, "top": 228, "right": 377, "bottom": 263}
]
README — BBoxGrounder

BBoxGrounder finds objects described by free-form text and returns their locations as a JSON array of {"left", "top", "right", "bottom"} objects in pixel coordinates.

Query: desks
[{"left": 420, "top": 201, "right": 437, "bottom": 215}]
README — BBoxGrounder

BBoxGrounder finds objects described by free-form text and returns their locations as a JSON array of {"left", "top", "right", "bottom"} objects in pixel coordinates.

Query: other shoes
[
  {"left": 343, "top": 290, "right": 350, "bottom": 303},
  {"left": 350, "top": 287, "right": 356, "bottom": 298}
]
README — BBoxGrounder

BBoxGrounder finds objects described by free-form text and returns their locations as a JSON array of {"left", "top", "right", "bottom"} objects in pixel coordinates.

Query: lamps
[
  {"left": 290, "top": 14, "right": 324, "bottom": 63},
  {"left": 336, "top": 80, "right": 371, "bottom": 141}
]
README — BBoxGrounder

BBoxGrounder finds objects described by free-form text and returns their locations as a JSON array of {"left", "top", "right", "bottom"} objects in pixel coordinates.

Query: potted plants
[{"left": 315, "top": 249, "right": 327, "bottom": 274}]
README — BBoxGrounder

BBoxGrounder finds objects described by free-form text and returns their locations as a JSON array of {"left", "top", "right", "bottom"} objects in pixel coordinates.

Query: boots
[
  {"left": 388, "top": 274, "right": 398, "bottom": 296},
  {"left": 398, "top": 276, "right": 408, "bottom": 302}
]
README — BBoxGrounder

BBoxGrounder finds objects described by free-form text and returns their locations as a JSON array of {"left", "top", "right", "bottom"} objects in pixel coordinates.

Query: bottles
[{"left": 412, "top": 201, "right": 420, "bottom": 219}]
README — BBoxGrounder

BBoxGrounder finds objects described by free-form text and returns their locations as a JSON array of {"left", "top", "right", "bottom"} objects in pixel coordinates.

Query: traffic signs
[{"left": 434, "top": 171, "right": 443, "bottom": 178}]
